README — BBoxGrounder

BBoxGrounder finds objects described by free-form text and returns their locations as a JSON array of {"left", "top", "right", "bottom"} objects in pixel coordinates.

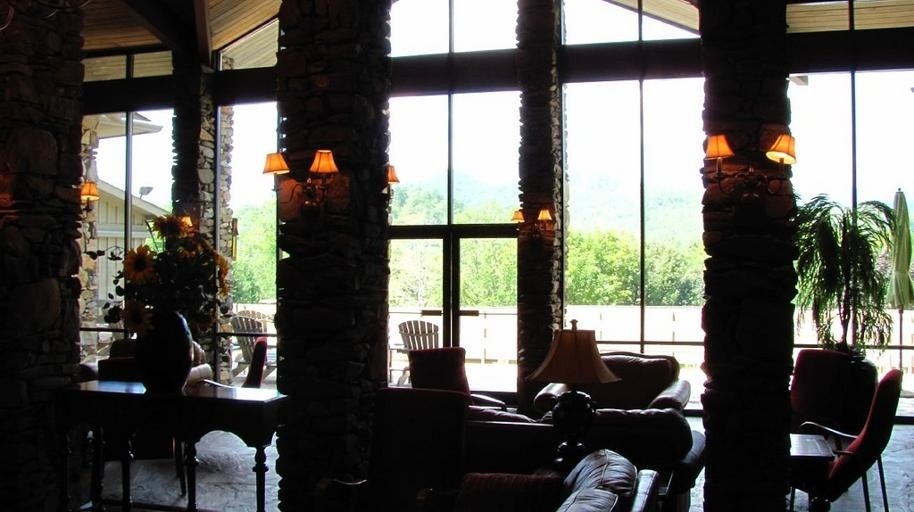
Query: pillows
[
  {"left": 556, "top": 489, "right": 617, "bottom": 512},
  {"left": 565, "top": 447, "right": 639, "bottom": 497}
]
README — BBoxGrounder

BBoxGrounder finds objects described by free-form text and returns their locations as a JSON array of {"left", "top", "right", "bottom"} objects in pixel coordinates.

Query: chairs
[
  {"left": 789, "top": 372, "right": 902, "bottom": 512},
  {"left": 407, "top": 348, "right": 508, "bottom": 408},
  {"left": 242, "top": 341, "right": 265, "bottom": 384},
  {"left": 396, "top": 317, "right": 440, "bottom": 385},
  {"left": 97, "top": 307, "right": 194, "bottom": 498},
  {"left": 790, "top": 353, "right": 877, "bottom": 432},
  {"left": 366, "top": 395, "right": 480, "bottom": 500}
]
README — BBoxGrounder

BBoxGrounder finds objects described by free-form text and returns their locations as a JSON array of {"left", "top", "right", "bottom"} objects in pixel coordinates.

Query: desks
[
  {"left": 789, "top": 433, "right": 834, "bottom": 459},
  {"left": 59, "top": 375, "right": 290, "bottom": 512},
  {"left": 467, "top": 418, "right": 558, "bottom": 447}
]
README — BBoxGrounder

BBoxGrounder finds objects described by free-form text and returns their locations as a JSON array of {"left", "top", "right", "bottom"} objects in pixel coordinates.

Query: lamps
[
  {"left": 79, "top": 179, "right": 95, "bottom": 198},
  {"left": 267, "top": 149, "right": 340, "bottom": 207},
  {"left": 526, "top": 320, "right": 621, "bottom": 424},
  {"left": 513, "top": 206, "right": 555, "bottom": 235},
  {"left": 703, "top": 132, "right": 795, "bottom": 204}
]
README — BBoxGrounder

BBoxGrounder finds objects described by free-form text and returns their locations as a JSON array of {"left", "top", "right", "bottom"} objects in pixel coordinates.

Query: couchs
[
  {"left": 451, "top": 451, "right": 660, "bottom": 512},
  {"left": 533, "top": 355, "right": 686, "bottom": 413},
  {"left": 576, "top": 404, "right": 699, "bottom": 460}
]
